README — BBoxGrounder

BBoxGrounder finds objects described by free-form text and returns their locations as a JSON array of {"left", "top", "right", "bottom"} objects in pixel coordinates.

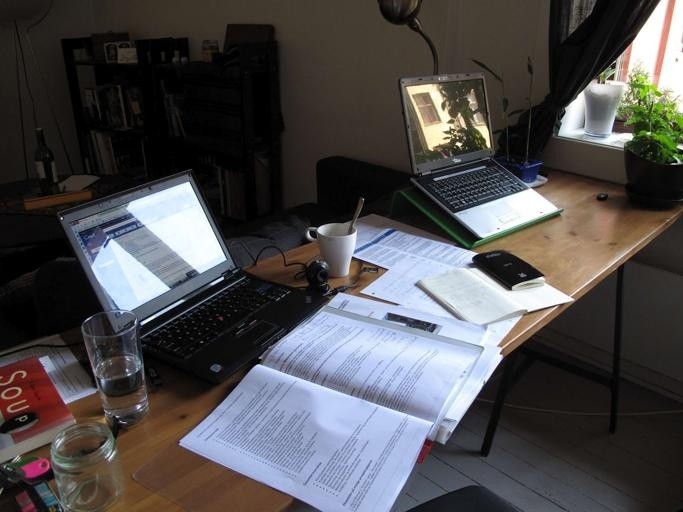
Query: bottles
[{"left": 35, "top": 127, "right": 59, "bottom": 186}]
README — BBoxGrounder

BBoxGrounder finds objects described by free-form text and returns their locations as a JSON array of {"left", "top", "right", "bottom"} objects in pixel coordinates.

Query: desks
[{"left": 23, "top": 170, "right": 683, "bottom": 512}]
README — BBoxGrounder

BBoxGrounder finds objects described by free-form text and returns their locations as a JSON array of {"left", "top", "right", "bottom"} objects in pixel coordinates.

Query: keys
[{"left": 0, "top": 453, "right": 52, "bottom": 495}]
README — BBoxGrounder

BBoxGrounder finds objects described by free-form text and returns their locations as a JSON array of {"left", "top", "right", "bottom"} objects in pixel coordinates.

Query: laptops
[
  {"left": 398, "top": 70, "right": 564, "bottom": 240},
  {"left": 57, "top": 168, "right": 330, "bottom": 387}
]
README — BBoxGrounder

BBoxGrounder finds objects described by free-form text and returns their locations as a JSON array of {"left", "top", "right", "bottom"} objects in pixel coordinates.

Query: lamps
[{"left": 376, "top": 1, "right": 439, "bottom": 77}]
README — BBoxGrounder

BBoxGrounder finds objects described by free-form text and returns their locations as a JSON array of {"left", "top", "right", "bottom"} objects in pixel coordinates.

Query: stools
[{"left": 405, "top": 486, "right": 522, "bottom": 512}]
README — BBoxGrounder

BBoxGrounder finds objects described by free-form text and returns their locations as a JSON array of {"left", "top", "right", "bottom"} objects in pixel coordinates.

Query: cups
[
  {"left": 304, "top": 223, "right": 357, "bottom": 277},
  {"left": 81, "top": 310, "right": 148, "bottom": 428},
  {"left": 49, "top": 422, "right": 125, "bottom": 512}
]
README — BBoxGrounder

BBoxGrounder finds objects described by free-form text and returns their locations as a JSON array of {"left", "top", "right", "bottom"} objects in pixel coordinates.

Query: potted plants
[
  {"left": 616, "top": 75, "right": 683, "bottom": 207},
  {"left": 472, "top": 53, "right": 544, "bottom": 182},
  {"left": 584, "top": 58, "right": 628, "bottom": 139}
]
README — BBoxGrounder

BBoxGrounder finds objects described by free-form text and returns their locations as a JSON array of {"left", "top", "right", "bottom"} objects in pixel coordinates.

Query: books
[
  {"left": 1, "top": 479, "right": 66, "bottom": 512},
  {"left": 0, "top": 356, "right": 77, "bottom": 465},
  {"left": 414, "top": 263, "right": 575, "bottom": 326},
  {"left": 178, "top": 305, "right": 485, "bottom": 512},
  {"left": 81, "top": 77, "right": 273, "bottom": 223},
  {"left": 473, "top": 248, "right": 547, "bottom": 291}
]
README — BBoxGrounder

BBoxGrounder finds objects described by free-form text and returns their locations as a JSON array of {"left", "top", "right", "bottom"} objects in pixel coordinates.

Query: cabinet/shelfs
[{"left": 59, "top": 18, "right": 285, "bottom": 235}]
